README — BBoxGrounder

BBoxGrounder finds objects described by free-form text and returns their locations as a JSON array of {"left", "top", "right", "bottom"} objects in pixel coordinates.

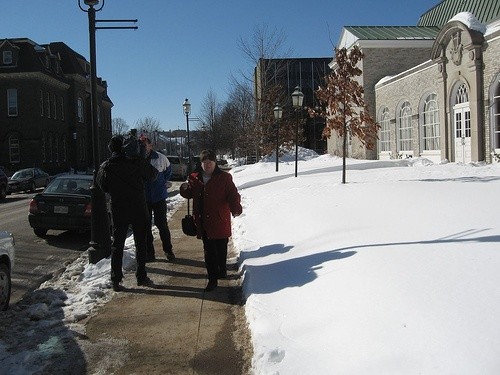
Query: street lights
[
  {"left": 72, "top": 132, "right": 79, "bottom": 174},
  {"left": 272, "top": 102, "right": 283, "bottom": 171},
  {"left": 183, "top": 98, "right": 192, "bottom": 164},
  {"left": 291, "top": 85, "right": 305, "bottom": 177}
]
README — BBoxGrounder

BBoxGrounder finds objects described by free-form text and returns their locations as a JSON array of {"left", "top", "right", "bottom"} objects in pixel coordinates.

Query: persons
[
  {"left": 137, "top": 133, "right": 176, "bottom": 262},
  {"left": 180, "top": 149, "right": 243, "bottom": 291},
  {"left": 96, "top": 134, "right": 159, "bottom": 292}
]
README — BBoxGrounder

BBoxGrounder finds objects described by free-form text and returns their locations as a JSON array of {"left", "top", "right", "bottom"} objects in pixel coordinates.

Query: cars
[
  {"left": 0, "top": 167, "right": 9, "bottom": 202},
  {"left": 27, "top": 173, "right": 114, "bottom": 240},
  {"left": 6, "top": 167, "right": 51, "bottom": 195},
  {"left": 0, "top": 229, "right": 16, "bottom": 312}
]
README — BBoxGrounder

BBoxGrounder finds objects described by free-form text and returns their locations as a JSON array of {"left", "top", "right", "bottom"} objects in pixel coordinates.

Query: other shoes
[
  {"left": 136, "top": 277, "right": 154, "bottom": 286},
  {"left": 145, "top": 256, "right": 157, "bottom": 263},
  {"left": 111, "top": 275, "right": 121, "bottom": 291},
  {"left": 165, "top": 251, "right": 176, "bottom": 258},
  {"left": 206, "top": 278, "right": 218, "bottom": 291}
]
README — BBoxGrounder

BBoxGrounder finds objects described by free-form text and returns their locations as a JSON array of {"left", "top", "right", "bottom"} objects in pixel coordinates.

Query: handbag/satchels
[{"left": 181, "top": 215, "right": 198, "bottom": 235}]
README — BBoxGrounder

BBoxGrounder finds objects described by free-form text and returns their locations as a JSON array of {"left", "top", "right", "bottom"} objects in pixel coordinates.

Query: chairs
[{"left": 67, "top": 181, "right": 77, "bottom": 190}]
